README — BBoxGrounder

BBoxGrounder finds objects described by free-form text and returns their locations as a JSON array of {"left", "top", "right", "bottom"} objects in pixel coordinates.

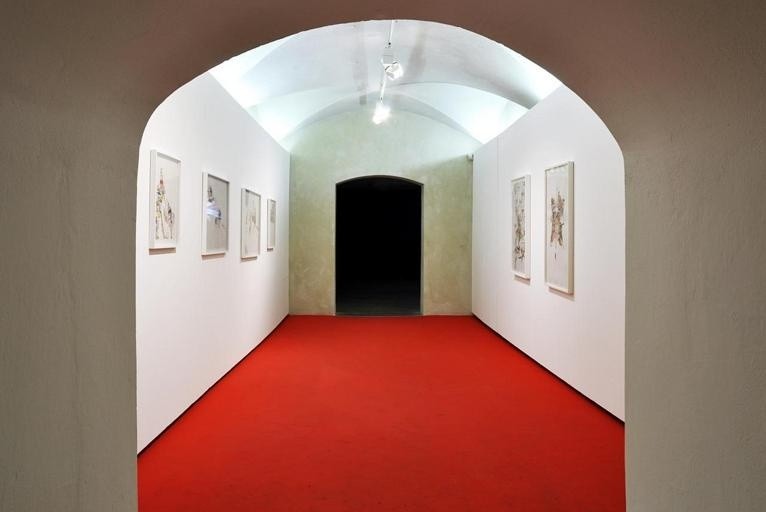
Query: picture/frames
[
  {"left": 239, "top": 187, "right": 279, "bottom": 260},
  {"left": 201, "top": 169, "right": 231, "bottom": 258},
  {"left": 148, "top": 148, "right": 181, "bottom": 251},
  {"left": 543, "top": 161, "right": 575, "bottom": 295},
  {"left": 509, "top": 173, "right": 531, "bottom": 281}
]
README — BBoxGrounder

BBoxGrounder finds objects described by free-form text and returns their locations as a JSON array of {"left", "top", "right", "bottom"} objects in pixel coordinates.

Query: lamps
[{"left": 371, "top": 19, "right": 407, "bottom": 127}]
